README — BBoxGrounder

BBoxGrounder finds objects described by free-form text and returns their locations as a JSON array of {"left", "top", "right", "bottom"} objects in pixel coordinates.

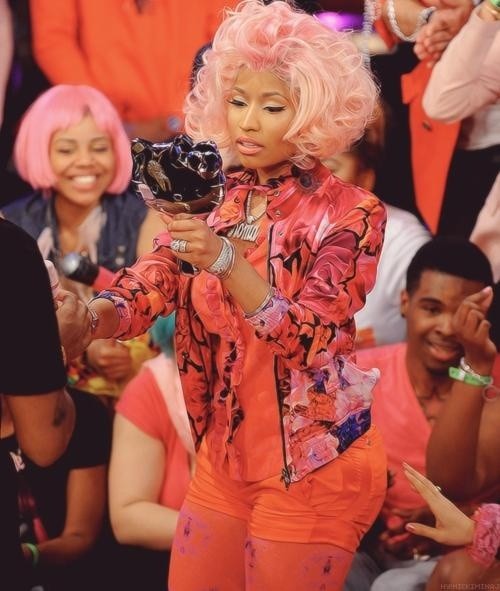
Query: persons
[{"left": 1, "top": 1, "right": 500, "bottom": 590}]
